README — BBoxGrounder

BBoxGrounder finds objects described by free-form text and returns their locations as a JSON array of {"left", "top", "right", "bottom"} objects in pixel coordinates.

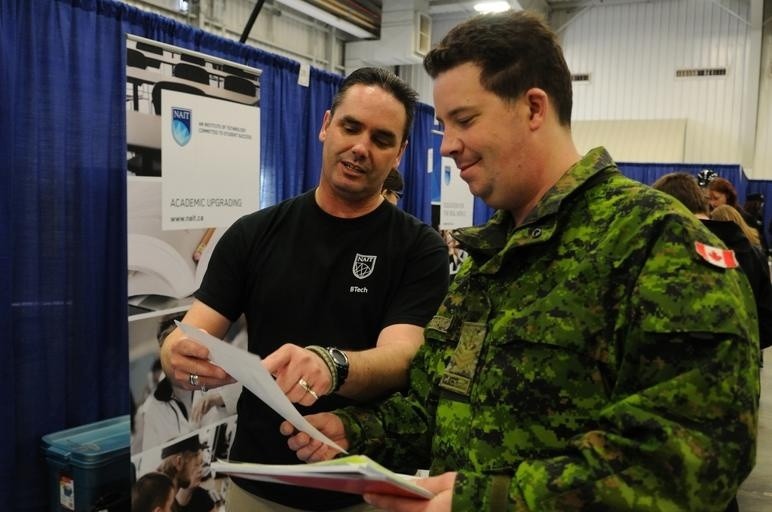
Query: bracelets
[{"left": 305, "top": 345, "right": 338, "bottom": 396}]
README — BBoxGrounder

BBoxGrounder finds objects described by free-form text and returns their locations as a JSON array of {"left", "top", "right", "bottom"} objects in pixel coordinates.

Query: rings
[
  {"left": 298, "top": 376, "right": 311, "bottom": 393},
  {"left": 310, "top": 389, "right": 319, "bottom": 402}
]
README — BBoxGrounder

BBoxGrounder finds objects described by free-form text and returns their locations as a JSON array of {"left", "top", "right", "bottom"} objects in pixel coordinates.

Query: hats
[
  {"left": 162, "top": 434, "right": 210, "bottom": 460},
  {"left": 384, "top": 168, "right": 404, "bottom": 198}
]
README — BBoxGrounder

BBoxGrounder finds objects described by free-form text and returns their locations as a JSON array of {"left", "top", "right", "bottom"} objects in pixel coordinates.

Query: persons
[
  {"left": 704, "top": 175, "right": 770, "bottom": 260},
  {"left": 381, "top": 166, "right": 405, "bottom": 206},
  {"left": 744, "top": 189, "right": 766, "bottom": 232},
  {"left": 279, "top": 7, "right": 762, "bottom": 511},
  {"left": 650, "top": 172, "right": 772, "bottom": 511},
  {"left": 158, "top": 65, "right": 450, "bottom": 512},
  {"left": 709, "top": 201, "right": 761, "bottom": 253},
  {"left": 128, "top": 312, "right": 238, "bottom": 512}
]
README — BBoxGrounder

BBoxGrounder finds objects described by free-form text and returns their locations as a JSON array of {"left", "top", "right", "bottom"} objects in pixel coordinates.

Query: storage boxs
[{"left": 41, "top": 413, "right": 131, "bottom": 512}]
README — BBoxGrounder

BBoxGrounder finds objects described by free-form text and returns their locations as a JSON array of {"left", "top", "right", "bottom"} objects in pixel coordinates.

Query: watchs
[{"left": 323, "top": 347, "right": 351, "bottom": 397}]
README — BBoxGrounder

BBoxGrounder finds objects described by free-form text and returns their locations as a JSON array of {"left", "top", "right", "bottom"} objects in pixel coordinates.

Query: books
[
  {"left": 124, "top": 226, "right": 231, "bottom": 302},
  {"left": 208, "top": 448, "right": 436, "bottom": 503}
]
